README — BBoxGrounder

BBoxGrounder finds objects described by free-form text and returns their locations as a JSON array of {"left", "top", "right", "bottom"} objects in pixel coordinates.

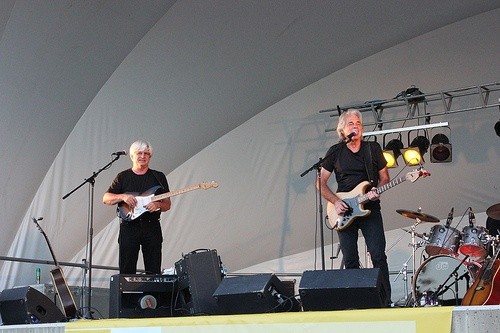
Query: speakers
[
  {"left": 299, "top": 267, "right": 392, "bottom": 312},
  {"left": 174, "top": 250, "right": 222, "bottom": 316},
  {"left": 0, "top": 286, "right": 67, "bottom": 325},
  {"left": 108, "top": 274, "right": 186, "bottom": 318},
  {"left": 212, "top": 273, "right": 302, "bottom": 315}
]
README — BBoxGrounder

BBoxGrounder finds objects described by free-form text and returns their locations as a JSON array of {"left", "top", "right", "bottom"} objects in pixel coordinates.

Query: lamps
[
  {"left": 429, "top": 127, "right": 452, "bottom": 163},
  {"left": 400, "top": 129, "right": 429, "bottom": 166},
  {"left": 382, "top": 132, "right": 404, "bottom": 168}
]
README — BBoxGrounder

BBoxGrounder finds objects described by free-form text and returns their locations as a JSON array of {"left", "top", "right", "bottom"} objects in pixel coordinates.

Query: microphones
[
  {"left": 112, "top": 150, "right": 128, "bottom": 156},
  {"left": 469, "top": 210, "right": 475, "bottom": 228},
  {"left": 444, "top": 211, "right": 454, "bottom": 228},
  {"left": 347, "top": 129, "right": 359, "bottom": 138}
]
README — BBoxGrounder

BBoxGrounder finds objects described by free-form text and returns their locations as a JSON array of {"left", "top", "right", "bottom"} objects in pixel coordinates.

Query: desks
[{"left": 14, "top": 283, "right": 93, "bottom": 318}]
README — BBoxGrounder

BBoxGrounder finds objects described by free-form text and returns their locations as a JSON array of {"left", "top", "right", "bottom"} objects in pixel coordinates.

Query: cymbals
[
  {"left": 396, "top": 208, "right": 440, "bottom": 224},
  {"left": 400, "top": 227, "right": 426, "bottom": 241},
  {"left": 486, "top": 203, "right": 500, "bottom": 221}
]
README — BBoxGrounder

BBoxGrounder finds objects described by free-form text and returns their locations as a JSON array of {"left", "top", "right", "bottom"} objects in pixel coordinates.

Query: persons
[
  {"left": 316, "top": 110, "right": 395, "bottom": 307},
  {"left": 103, "top": 141, "right": 170, "bottom": 274}
]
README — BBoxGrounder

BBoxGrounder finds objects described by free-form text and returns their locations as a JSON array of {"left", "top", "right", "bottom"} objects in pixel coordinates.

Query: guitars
[
  {"left": 31, "top": 216, "right": 79, "bottom": 319},
  {"left": 117, "top": 179, "right": 220, "bottom": 222},
  {"left": 326, "top": 166, "right": 432, "bottom": 231},
  {"left": 460, "top": 246, "right": 500, "bottom": 306}
]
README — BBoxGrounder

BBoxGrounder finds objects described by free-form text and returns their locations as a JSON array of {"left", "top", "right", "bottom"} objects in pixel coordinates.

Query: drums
[
  {"left": 458, "top": 225, "right": 490, "bottom": 257},
  {"left": 411, "top": 253, "right": 478, "bottom": 308},
  {"left": 424, "top": 224, "right": 461, "bottom": 257}
]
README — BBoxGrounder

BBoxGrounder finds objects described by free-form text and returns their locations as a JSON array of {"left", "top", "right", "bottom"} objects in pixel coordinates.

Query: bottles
[{"left": 221, "top": 264, "right": 226, "bottom": 279}]
